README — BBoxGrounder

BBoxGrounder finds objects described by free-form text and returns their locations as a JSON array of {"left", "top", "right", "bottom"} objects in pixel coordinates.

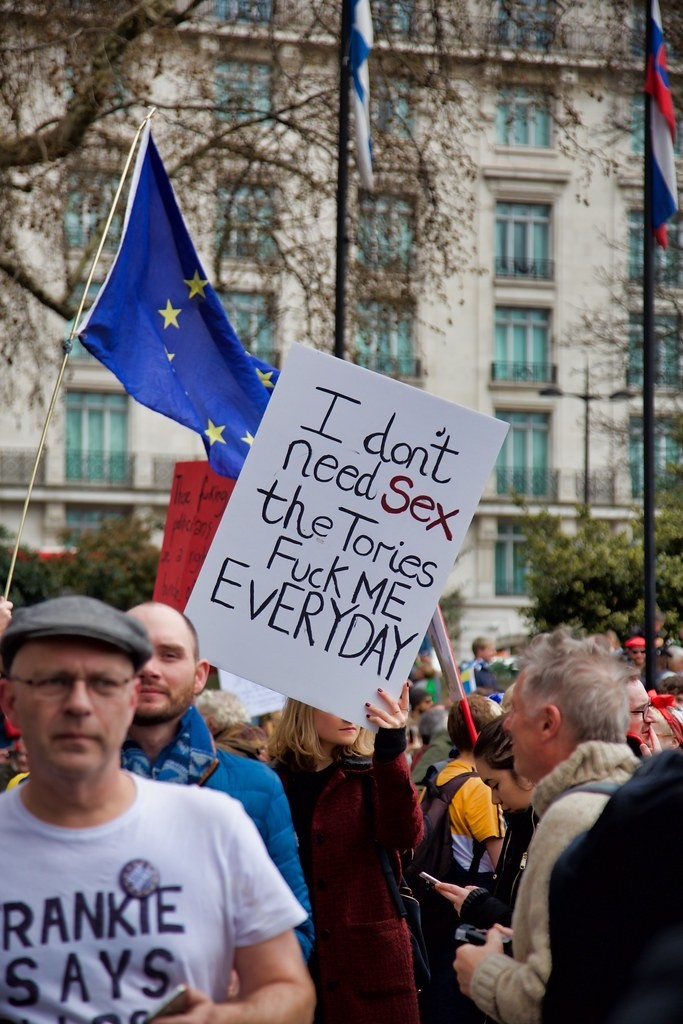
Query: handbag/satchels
[{"left": 400, "top": 885, "right": 431, "bottom": 990}]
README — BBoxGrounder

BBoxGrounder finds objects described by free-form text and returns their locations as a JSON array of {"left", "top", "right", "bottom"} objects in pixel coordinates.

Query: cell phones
[
  {"left": 419, "top": 872, "right": 442, "bottom": 885},
  {"left": 142, "top": 985, "right": 190, "bottom": 1024}
]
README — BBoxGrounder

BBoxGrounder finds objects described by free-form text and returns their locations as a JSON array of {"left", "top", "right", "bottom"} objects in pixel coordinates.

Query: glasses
[
  {"left": 633, "top": 650, "right": 646, "bottom": 654},
  {"left": 9, "top": 673, "right": 134, "bottom": 697},
  {"left": 630, "top": 703, "right": 649, "bottom": 721}
]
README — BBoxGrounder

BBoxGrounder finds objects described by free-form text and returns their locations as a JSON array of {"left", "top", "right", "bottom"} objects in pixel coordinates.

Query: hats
[
  {"left": 624, "top": 635, "right": 646, "bottom": 648},
  {"left": 0, "top": 594, "right": 153, "bottom": 672}
]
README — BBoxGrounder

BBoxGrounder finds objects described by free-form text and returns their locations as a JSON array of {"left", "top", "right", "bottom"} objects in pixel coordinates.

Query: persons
[{"left": 0, "top": 597, "right": 683, "bottom": 1022}]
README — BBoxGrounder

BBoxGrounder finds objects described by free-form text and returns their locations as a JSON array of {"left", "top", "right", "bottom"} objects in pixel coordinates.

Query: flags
[
  {"left": 348, "top": 1, "right": 374, "bottom": 190},
  {"left": 646, "top": 1, "right": 680, "bottom": 250},
  {"left": 74, "top": 126, "right": 281, "bottom": 480}
]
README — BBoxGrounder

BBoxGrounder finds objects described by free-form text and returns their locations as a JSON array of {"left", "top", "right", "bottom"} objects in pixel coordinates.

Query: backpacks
[{"left": 407, "top": 771, "right": 488, "bottom": 900}]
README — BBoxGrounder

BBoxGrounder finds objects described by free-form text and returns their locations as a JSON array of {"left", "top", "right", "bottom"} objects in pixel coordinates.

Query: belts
[{"left": 480, "top": 871, "right": 495, "bottom": 879}]
